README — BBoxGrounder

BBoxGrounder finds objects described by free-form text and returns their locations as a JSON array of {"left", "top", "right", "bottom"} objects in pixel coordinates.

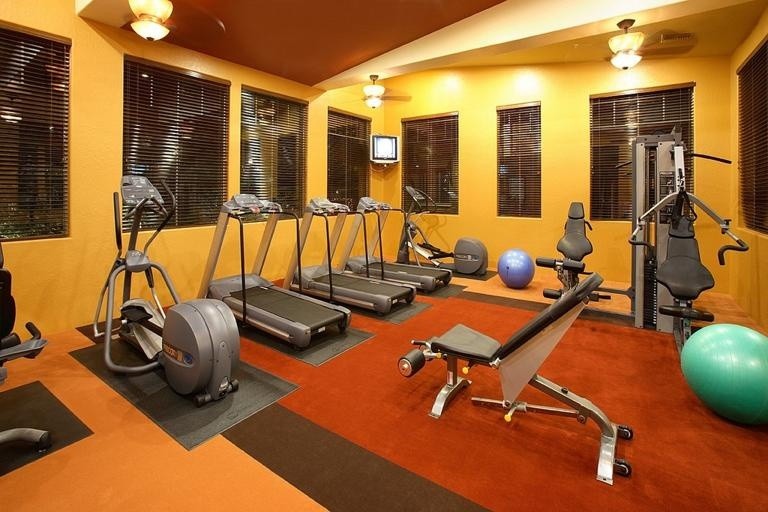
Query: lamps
[
  {"left": 126, "top": 0, "right": 172, "bottom": 44},
  {"left": 362, "top": 75, "right": 386, "bottom": 110},
  {"left": 606, "top": 19, "right": 646, "bottom": 71}
]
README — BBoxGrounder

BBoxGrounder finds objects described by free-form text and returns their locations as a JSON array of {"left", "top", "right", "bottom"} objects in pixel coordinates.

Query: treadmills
[
  {"left": 197, "top": 194, "right": 352, "bottom": 351},
  {"left": 336, "top": 196, "right": 453, "bottom": 295},
  {"left": 282, "top": 196, "right": 417, "bottom": 316}
]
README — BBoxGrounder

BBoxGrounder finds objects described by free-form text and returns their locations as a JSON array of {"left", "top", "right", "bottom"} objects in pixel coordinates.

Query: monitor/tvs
[{"left": 368, "top": 134, "right": 400, "bottom": 163}]
